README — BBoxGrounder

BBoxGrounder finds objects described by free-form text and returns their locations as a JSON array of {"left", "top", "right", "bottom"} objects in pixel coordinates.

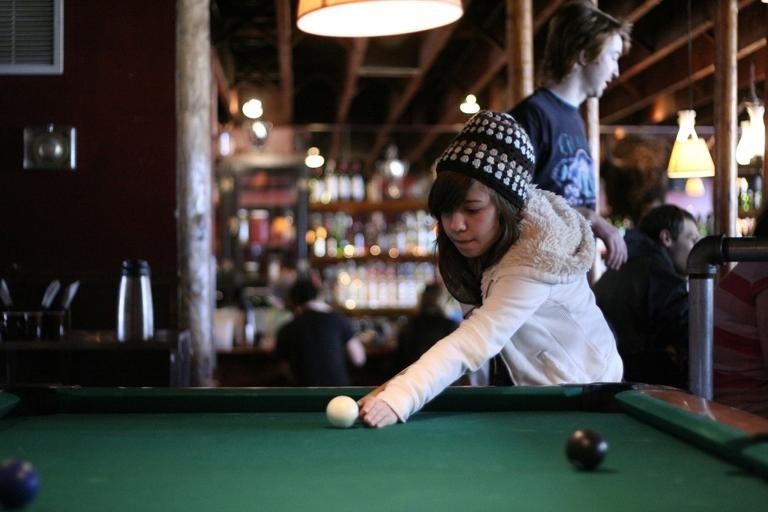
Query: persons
[
  {"left": 353, "top": 110, "right": 626, "bottom": 429},
  {"left": 261, "top": 279, "right": 368, "bottom": 387},
  {"left": 592, "top": 204, "right": 702, "bottom": 390},
  {"left": 710, "top": 203, "right": 767, "bottom": 423},
  {"left": 397, "top": 280, "right": 458, "bottom": 355},
  {"left": 507, "top": 1, "right": 630, "bottom": 273}
]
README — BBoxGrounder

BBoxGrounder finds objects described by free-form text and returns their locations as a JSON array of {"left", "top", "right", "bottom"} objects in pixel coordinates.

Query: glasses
[
  {"left": 665, "top": 0, "right": 714, "bottom": 181},
  {"left": 734, "top": 55, "right": 765, "bottom": 167},
  {"left": 294, "top": 1, "right": 468, "bottom": 43}
]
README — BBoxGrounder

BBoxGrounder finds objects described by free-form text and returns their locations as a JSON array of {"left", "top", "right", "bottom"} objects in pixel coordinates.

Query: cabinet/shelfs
[
  {"left": 606, "top": 178, "right": 762, "bottom": 243},
  {"left": 116, "top": 257, "right": 159, "bottom": 343}
]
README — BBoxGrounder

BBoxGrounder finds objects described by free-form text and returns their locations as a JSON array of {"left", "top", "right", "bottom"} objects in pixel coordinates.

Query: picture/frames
[{"left": 0, "top": 374, "right": 768, "bottom": 511}]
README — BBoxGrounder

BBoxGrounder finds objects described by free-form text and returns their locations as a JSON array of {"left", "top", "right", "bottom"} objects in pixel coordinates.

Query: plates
[{"left": 1, "top": 329, "right": 192, "bottom": 388}]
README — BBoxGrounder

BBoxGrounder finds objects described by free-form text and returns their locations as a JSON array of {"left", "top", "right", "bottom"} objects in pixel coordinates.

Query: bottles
[
  {"left": 213, "top": 145, "right": 465, "bottom": 353},
  {"left": 606, "top": 178, "right": 762, "bottom": 243},
  {"left": 116, "top": 257, "right": 159, "bottom": 343},
  {"left": 435, "top": 109, "right": 538, "bottom": 210}
]
